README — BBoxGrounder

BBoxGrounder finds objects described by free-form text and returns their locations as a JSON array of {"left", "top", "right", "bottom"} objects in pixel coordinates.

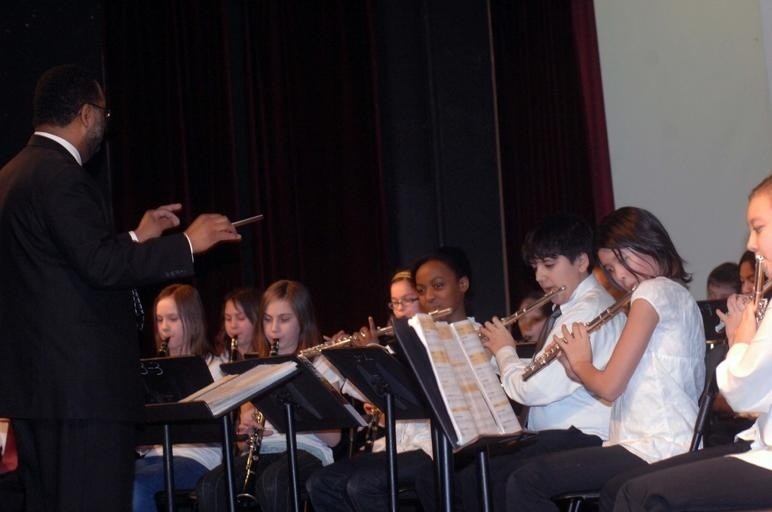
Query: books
[
  {"left": 176, "top": 361, "right": 296, "bottom": 416},
  {"left": 410, "top": 312, "right": 524, "bottom": 447}
]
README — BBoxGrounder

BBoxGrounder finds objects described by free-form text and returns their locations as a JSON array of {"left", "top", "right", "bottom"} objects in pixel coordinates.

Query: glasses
[
  {"left": 387, "top": 297, "right": 420, "bottom": 310},
  {"left": 84, "top": 101, "right": 112, "bottom": 119}
]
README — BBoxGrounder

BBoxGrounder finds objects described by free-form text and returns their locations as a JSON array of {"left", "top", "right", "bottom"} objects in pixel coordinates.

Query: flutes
[
  {"left": 476, "top": 286, "right": 566, "bottom": 339},
  {"left": 753, "top": 256, "right": 764, "bottom": 318},
  {"left": 522, "top": 286, "right": 640, "bottom": 383},
  {"left": 300, "top": 307, "right": 451, "bottom": 360}
]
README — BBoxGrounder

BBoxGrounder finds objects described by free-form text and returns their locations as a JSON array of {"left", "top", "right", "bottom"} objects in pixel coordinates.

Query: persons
[
  {"left": 600, "top": 169, "right": 772, "bottom": 512},
  {"left": 488, "top": 207, "right": 705, "bottom": 511},
  {"left": 515, "top": 290, "right": 553, "bottom": 342},
  {"left": 215, "top": 284, "right": 264, "bottom": 365},
  {"left": 383, "top": 268, "right": 427, "bottom": 322},
  {"left": 300, "top": 244, "right": 474, "bottom": 510},
  {"left": 193, "top": 279, "right": 342, "bottom": 511},
  {"left": 412, "top": 211, "right": 628, "bottom": 511},
  {"left": 705, "top": 259, "right": 741, "bottom": 301},
  {"left": 737, "top": 249, "right": 766, "bottom": 298},
  {"left": 0, "top": 63, "right": 245, "bottom": 511},
  {"left": 130, "top": 282, "right": 229, "bottom": 512}
]
